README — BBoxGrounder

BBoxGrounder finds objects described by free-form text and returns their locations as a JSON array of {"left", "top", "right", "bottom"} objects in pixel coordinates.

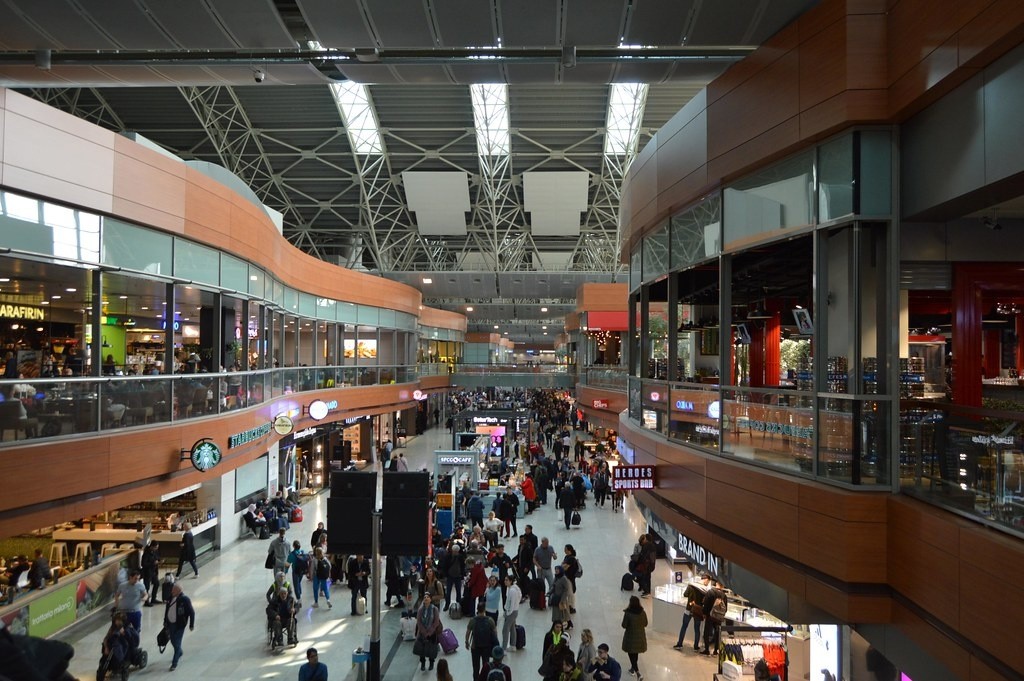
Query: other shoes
[
  {"left": 674, "top": 645, "right": 683, "bottom": 649},
  {"left": 700, "top": 650, "right": 710, "bottom": 655},
  {"left": 312, "top": 603, "right": 318, "bottom": 607},
  {"left": 289, "top": 639, "right": 298, "bottom": 644},
  {"left": 694, "top": 647, "right": 699, "bottom": 651},
  {"left": 429, "top": 663, "right": 434, "bottom": 670},
  {"left": 637, "top": 675, "right": 644, "bottom": 681},
  {"left": 145, "top": 603, "right": 153, "bottom": 607},
  {"left": 395, "top": 603, "right": 403, "bottom": 607},
  {"left": 421, "top": 663, "right": 425, "bottom": 671},
  {"left": 170, "top": 665, "right": 176, "bottom": 671},
  {"left": 327, "top": 600, "right": 332, "bottom": 607},
  {"left": 629, "top": 669, "right": 634, "bottom": 676},
  {"left": 152, "top": 600, "right": 161, "bottom": 604},
  {"left": 277, "top": 641, "right": 284, "bottom": 646},
  {"left": 385, "top": 602, "right": 390, "bottom": 606},
  {"left": 714, "top": 652, "right": 718, "bottom": 656}
]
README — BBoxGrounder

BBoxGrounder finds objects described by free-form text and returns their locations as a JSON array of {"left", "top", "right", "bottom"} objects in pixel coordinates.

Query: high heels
[{"left": 565, "top": 623, "right": 573, "bottom": 630}]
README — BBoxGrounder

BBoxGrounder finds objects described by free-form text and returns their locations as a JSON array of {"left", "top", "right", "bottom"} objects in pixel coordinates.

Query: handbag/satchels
[
  {"left": 266, "top": 553, "right": 274, "bottom": 569},
  {"left": 356, "top": 592, "right": 366, "bottom": 615},
  {"left": 692, "top": 601, "right": 704, "bottom": 621},
  {"left": 449, "top": 602, "right": 463, "bottom": 619},
  {"left": 157, "top": 628, "right": 169, "bottom": 647},
  {"left": 399, "top": 610, "right": 418, "bottom": 640},
  {"left": 572, "top": 510, "right": 581, "bottom": 525}
]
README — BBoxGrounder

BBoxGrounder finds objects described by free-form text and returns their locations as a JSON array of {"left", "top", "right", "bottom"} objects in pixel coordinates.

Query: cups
[{"left": 994, "top": 376, "right": 1024, "bottom": 385}]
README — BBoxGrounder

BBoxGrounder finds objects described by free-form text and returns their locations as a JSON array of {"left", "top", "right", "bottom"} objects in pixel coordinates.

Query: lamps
[
  {"left": 676, "top": 271, "right": 776, "bottom": 333},
  {"left": 302, "top": 399, "right": 328, "bottom": 421}
]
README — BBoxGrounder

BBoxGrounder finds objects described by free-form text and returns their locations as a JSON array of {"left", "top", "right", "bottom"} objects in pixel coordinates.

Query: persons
[
  {"left": 0, "top": 330, "right": 727, "bottom": 681},
  {"left": 1007, "top": 366, "right": 1019, "bottom": 379},
  {"left": 980, "top": 355, "right": 988, "bottom": 379}
]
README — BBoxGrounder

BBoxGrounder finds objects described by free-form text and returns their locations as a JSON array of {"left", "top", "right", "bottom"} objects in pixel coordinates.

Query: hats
[{"left": 491, "top": 646, "right": 504, "bottom": 658}]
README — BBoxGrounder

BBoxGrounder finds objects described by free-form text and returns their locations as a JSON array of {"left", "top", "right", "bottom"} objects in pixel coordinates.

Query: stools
[
  {"left": 48, "top": 542, "right": 69, "bottom": 567},
  {"left": 74, "top": 543, "right": 92, "bottom": 567},
  {"left": 101, "top": 543, "right": 116, "bottom": 558},
  {"left": 120, "top": 543, "right": 135, "bottom": 550},
  {"left": 762, "top": 393, "right": 794, "bottom": 451}
]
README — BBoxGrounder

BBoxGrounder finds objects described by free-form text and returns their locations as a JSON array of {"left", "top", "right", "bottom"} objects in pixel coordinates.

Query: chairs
[
  {"left": 100, "top": 394, "right": 126, "bottom": 429},
  {"left": 728, "top": 393, "right": 754, "bottom": 439},
  {"left": 180, "top": 387, "right": 209, "bottom": 419},
  {"left": 6, "top": 567, "right": 31, "bottom": 594},
  {"left": 128, "top": 391, "right": 170, "bottom": 425},
  {"left": 0, "top": 400, "right": 38, "bottom": 441}
]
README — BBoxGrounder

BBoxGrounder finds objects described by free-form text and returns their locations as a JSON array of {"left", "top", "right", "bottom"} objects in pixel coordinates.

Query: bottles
[{"left": 1008, "top": 365, "right": 1018, "bottom": 378}]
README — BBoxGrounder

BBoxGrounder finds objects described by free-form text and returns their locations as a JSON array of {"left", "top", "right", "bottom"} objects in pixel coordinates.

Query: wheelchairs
[
  {"left": 101, "top": 609, "right": 148, "bottom": 681},
  {"left": 266, "top": 605, "right": 299, "bottom": 652}
]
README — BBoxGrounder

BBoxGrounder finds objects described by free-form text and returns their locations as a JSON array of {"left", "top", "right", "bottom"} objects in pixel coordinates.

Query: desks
[
  {"left": 38, "top": 397, "right": 97, "bottom": 431},
  {"left": 946, "top": 425, "right": 1024, "bottom": 512}
]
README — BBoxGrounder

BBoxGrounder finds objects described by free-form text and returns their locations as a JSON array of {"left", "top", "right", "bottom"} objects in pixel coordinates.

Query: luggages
[
  {"left": 439, "top": 628, "right": 459, "bottom": 653},
  {"left": 529, "top": 571, "right": 546, "bottom": 610},
  {"left": 510, "top": 624, "right": 525, "bottom": 649}
]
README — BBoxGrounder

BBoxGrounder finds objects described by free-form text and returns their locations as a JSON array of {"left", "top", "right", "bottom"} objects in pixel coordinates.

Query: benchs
[{"left": 240, "top": 502, "right": 288, "bottom": 539}]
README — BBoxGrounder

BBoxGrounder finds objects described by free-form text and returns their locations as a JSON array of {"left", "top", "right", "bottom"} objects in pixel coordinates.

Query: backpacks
[
  {"left": 567, "top": 555, "right": 583, "bottom": 577},
  {"left": 315, "top": 558, "right": 330, "bottom": 579},
  {"left": 473, "top": 617, "right": 494, "bottom": 652},
  {"left": 622, "top": 573, "right": 634, "bottom": 590},
  {"left": 292, "top": 551, "right": 309, "bottom": 574},
  {"left": 486, "top": 662, "right": 506, "bottom": 681},
  {"left": 710, "top": 592, "right": 726, "bottom": 621}
]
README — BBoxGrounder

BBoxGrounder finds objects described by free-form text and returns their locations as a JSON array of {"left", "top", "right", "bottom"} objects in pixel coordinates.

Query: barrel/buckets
[{"left": 796, "top": 357, "right": 943, "bottom": 478}]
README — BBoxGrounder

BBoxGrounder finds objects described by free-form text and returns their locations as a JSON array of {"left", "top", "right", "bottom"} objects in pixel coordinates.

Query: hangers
[{"left": 722, "top": 635, "right": 785, "bottom": 646}]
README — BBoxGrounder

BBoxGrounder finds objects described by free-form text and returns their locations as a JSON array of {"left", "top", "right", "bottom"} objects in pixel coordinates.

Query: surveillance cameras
[{"left": 254, "top": 72, "right": 264, "bottom": 82}]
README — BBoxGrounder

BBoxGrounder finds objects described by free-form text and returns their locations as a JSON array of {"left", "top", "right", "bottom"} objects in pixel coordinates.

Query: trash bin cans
[{"left": 352, "top": 648, "right": 371, "bottom": 681}]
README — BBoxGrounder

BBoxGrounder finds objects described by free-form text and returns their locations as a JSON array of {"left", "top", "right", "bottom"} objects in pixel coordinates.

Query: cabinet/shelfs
[{"left": 82, "top": 496, "right": 197, "bottom": 530}]
